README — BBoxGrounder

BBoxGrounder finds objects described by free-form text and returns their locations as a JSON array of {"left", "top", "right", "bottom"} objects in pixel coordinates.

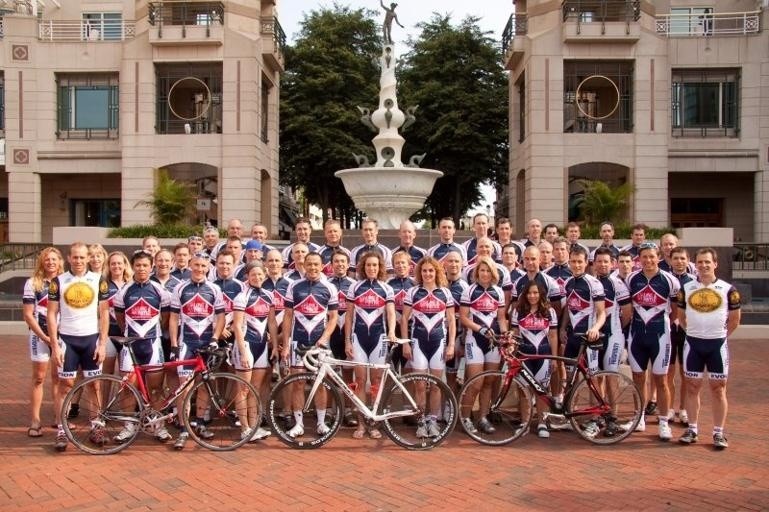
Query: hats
[{"left": 246, "top": 239, "right": 262, "bottom": 252}]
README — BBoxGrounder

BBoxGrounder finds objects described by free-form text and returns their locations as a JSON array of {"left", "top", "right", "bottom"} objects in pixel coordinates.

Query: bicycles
[
  {"left": 457, "top": 328, "right": 646, "bottom": 447},
  {"left": 265, "top": 339, "right": 457, "bottom": 451},
  {"left": 60, "top": 328, "right": 263, "bottom": 456}
]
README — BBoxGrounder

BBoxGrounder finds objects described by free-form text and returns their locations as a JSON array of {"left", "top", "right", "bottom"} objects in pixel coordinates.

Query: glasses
[
  {"left": 194, "top": 252, "right": 212, "bottom": 261},
  {"left": 133, "top": 248, "right": 152, "bottom": 255},
  {"left": 640, "top": 242, "right": 657, "bottom": 249},
  {"left": 189, "top": 235, "right": 203, "bottom": 243}
]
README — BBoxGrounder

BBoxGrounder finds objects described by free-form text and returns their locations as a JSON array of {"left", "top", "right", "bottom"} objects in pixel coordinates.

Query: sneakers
[
  {"left": 581, "top": 418, "right": 617, "bottom": 439},
  {"left": 620, "top": 417, "right": 646, "bottom": 432},
  {"left": 283, "top": 414, "right": 334, "bottom": 440},
  {"left": 645, "top": 402, "right": 657, "bottom": 417},
  {"left": 54, "top": 430, "right": 71, "bottom": 450},
  {"left": 460, "top": 415, "right": 495, "bottom": 436},
  {"left": 155, "top": 417, "right": 216, "bottom": 450},
  {"left": 656, "top": 410, "right": 689, "bottom": 441},
  {"left": 679, "top": 428, "right": 698, "bottom": 445},
  {"left": 241, "top": 426, "right": 273, "bottom": 443},
  {"left": 68, "top": 404, "right": 80, "bottom": 419},
  {"left": 325, "top": 409, "right": 358, "bottom": 426},
  {"left": 516, "top": 422, "right": 550, "bottom": 439},
  {"left": 415, "top": 415, "right": 442, "bottom": 438},
  {"left": 351, "top": 428, "right": 382, "bottom": 441},
  {"left": 88, "top": 419, "right": 135, "bottom": 445},
  {"left": 712, "top": 430, "right": 729, "bottom": 447}
]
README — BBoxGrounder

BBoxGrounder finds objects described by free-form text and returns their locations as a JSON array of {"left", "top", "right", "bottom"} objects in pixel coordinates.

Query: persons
[
  {"left": 24, "top": 214, "right": 403, "bottom": 450},
  {"left": 383, "top": 214, "right": 744, "bottom": 448},
  {"left": 380, "top": 0, "right": 403, "bottom": 45}
]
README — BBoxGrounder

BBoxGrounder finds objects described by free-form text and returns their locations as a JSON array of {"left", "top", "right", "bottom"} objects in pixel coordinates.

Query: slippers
[{"left": 25, "top": 425, "right": 45, "bottom": 437}]
